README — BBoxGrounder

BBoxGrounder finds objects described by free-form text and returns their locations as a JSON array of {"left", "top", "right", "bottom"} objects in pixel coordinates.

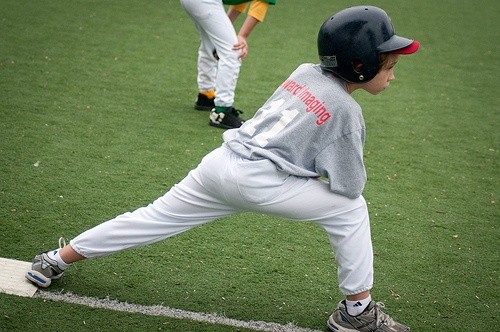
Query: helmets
[{"left": 317, "top": 5, "right": 414, "bottom": 84}]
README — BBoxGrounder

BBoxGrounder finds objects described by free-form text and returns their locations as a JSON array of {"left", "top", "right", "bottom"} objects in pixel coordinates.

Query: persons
[
  {"left": 26, "top": 6, "right": 420, "bottom": 332},
  {"left": 178, "top": 0, "right": 279, "bottom": 129}
]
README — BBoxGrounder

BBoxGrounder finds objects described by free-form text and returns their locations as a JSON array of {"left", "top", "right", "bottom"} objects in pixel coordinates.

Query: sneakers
[
  {"left": 209, "top": 107, "right": 246, "bottom": 129},
  {"left": 327, "top": 299, "right": 410, "bottom": 332},
  {"left": 195, "top": 92, "right": 215, "bottom": 111},
  {"left": 26, "top": 237, "right": 66, "bottom": 287}
]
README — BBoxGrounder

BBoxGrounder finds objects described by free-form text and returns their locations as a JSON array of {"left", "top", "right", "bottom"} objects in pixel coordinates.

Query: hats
[{"left": 391, "top": 40, "right": 419, "bottom": 54}]
